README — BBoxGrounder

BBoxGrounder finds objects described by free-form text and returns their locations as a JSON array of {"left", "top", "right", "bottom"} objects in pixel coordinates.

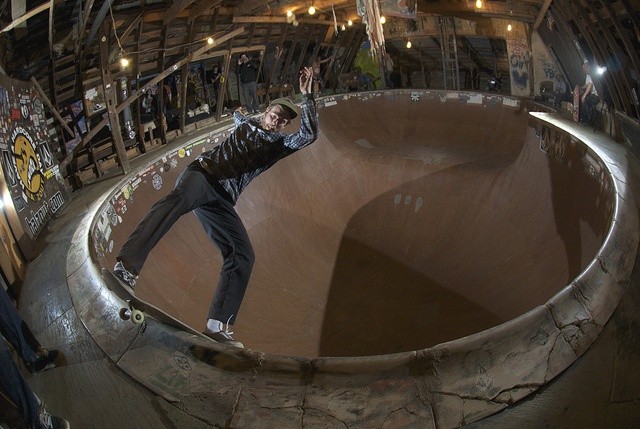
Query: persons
[{"left": 111, "top": 66, "right": 317, "bottom": 349}]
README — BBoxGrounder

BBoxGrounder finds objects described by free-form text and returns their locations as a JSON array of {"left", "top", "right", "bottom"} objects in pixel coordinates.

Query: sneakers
[
  {"left": 39, "top": 407, "right": 70, "bottom": 429},
  {"left": 201, "top": 314, "right": 244, "bottom": 349},
  {"left": 24, "top": 349, "right": 65, "bottom": 376},
  {"left": 112, "top": 260, "right": 138, "bottom": 287}
]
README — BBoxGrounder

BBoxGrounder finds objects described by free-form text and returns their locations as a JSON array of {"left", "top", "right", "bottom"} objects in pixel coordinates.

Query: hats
[{"left": 269, "top": 97, "right": 298, "bottom": 119}]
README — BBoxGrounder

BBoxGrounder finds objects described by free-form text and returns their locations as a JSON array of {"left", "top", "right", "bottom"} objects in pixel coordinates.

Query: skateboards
[
  {"left": 102, "top": 268, "right": 218, "bottom": 343},
  {"left": 571, "top": 85, "right": 579, "bottom": 122}
]
